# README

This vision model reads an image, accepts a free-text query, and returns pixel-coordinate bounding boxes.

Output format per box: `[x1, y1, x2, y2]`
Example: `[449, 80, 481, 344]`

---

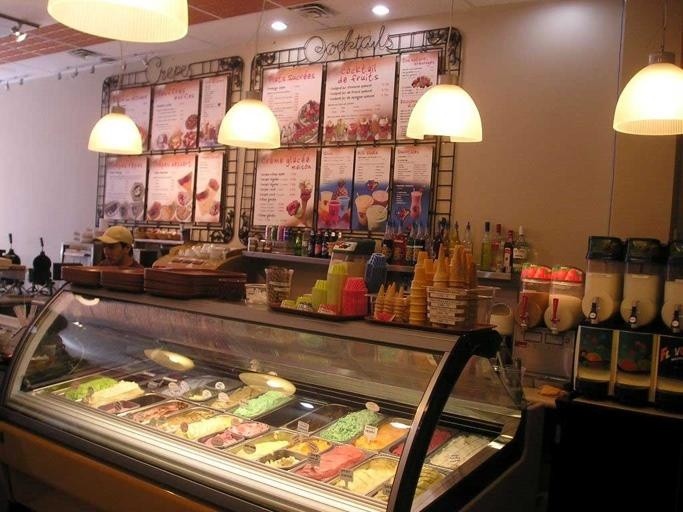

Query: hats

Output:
[92, 226, 133, 247]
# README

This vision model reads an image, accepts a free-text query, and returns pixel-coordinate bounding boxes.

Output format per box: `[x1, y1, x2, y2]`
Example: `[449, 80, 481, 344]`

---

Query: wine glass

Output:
[299, 183, 312, 223]
[178, 170, 192, 196]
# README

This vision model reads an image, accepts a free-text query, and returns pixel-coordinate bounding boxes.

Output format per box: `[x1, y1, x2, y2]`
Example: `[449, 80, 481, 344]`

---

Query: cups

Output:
[324, 115, 392, 140]
[319, 188, 349, 216]
[409, 190, 422, 219]
[264, 263, 294, 309]
[355, 190, 387, 231]
[198, 179, 219, 222]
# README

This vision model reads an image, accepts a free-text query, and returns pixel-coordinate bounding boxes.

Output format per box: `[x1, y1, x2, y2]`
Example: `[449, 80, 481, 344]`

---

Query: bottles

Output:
[379, 219, 528, 272]
[265, 222, 342, 260]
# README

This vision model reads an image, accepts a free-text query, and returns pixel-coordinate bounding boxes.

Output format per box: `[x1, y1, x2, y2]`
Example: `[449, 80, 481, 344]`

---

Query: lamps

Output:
[45, 0, 285, 158]
[10, 22, 28, 44]
[405, 2, 483, 144]
[612, 1, 683, 137]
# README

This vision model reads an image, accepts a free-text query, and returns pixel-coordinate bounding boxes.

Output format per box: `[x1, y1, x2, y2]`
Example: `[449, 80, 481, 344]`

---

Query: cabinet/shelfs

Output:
[59, 240, 104, 268]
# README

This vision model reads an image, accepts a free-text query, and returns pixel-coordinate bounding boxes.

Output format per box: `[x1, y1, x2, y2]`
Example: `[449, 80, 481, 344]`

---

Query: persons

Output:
[91, 224, 145, 270]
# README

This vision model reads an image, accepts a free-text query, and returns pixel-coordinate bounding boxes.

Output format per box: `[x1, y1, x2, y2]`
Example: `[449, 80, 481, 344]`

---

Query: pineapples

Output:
[336, 179, 349, 198]
[298, 181, 311, 213]
[285, 201, 308, 227]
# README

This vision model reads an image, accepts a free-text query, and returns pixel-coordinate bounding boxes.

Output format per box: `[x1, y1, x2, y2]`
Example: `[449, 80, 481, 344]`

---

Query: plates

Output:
[100, 200, 192, 223]
[157, 112, 198, 152]
[281, 99, 320, 145]
